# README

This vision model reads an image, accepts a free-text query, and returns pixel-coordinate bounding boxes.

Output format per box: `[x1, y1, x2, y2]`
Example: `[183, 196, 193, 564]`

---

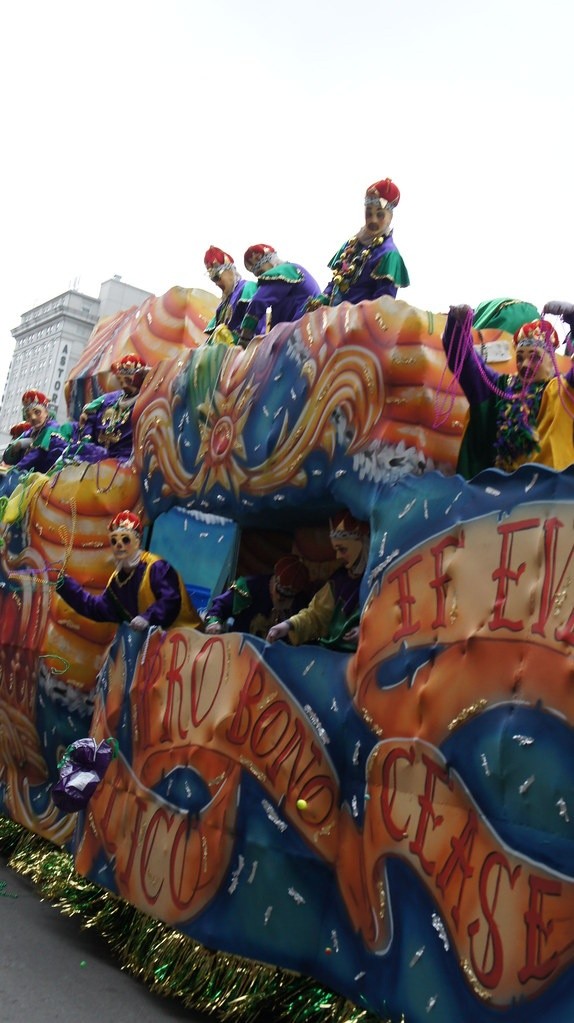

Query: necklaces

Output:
[0, 423, 129, 675]
[334, 234, 384, 293]
[106, 392, 138, 434]
[432, 308, 574, 430]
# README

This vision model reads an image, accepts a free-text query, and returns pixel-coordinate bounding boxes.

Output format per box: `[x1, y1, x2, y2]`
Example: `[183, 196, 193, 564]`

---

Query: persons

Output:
[55, 510, 205, 633]
[54, 356, 151, 465]
[306, 178, 410, 314]
[13, 392, 68, 475]
[237, 244, 321, 350]
[267, 515, 370, 655]
[203, 246, 266, 347]
[206, 554, 319, 647]
[442, 300, 574, 480]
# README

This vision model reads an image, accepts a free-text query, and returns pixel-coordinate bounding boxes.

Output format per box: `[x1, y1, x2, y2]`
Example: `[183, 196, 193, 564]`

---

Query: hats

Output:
[365, 177, 402, 212]
[514, 319, 561, 350]
[22, 390, 50, 407]
[204, 245, 235, 269]
[327, 514, 367, 541]
[273, 555, 311, 596]
[108, 511, 145, 533]
[110, 354, 145, 372]
[244, 244, 277, 272]
